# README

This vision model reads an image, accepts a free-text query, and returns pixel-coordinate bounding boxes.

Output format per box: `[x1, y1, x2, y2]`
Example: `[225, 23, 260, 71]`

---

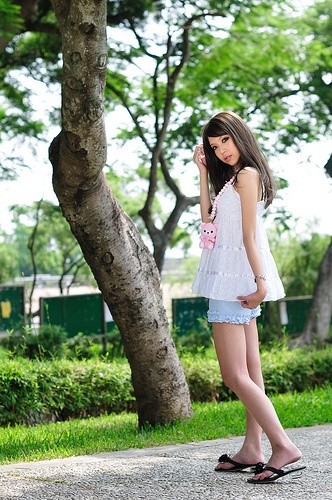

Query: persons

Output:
[192, 110, 308, 485]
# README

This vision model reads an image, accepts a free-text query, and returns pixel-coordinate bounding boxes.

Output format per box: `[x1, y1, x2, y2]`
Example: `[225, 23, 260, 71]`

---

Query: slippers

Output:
[214, 454, 266, 472]
[247, 465, 306, 483]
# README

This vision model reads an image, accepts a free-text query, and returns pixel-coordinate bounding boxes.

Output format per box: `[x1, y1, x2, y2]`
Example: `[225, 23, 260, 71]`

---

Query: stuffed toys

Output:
[199, 222, 219, 249]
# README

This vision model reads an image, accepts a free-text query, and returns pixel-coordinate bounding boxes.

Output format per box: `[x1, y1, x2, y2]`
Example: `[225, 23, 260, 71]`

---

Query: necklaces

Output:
[198, 169, 239, 249]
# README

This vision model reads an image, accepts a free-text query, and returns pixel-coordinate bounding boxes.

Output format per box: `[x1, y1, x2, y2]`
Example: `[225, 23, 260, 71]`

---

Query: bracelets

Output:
[253, 273, 267, 284]
